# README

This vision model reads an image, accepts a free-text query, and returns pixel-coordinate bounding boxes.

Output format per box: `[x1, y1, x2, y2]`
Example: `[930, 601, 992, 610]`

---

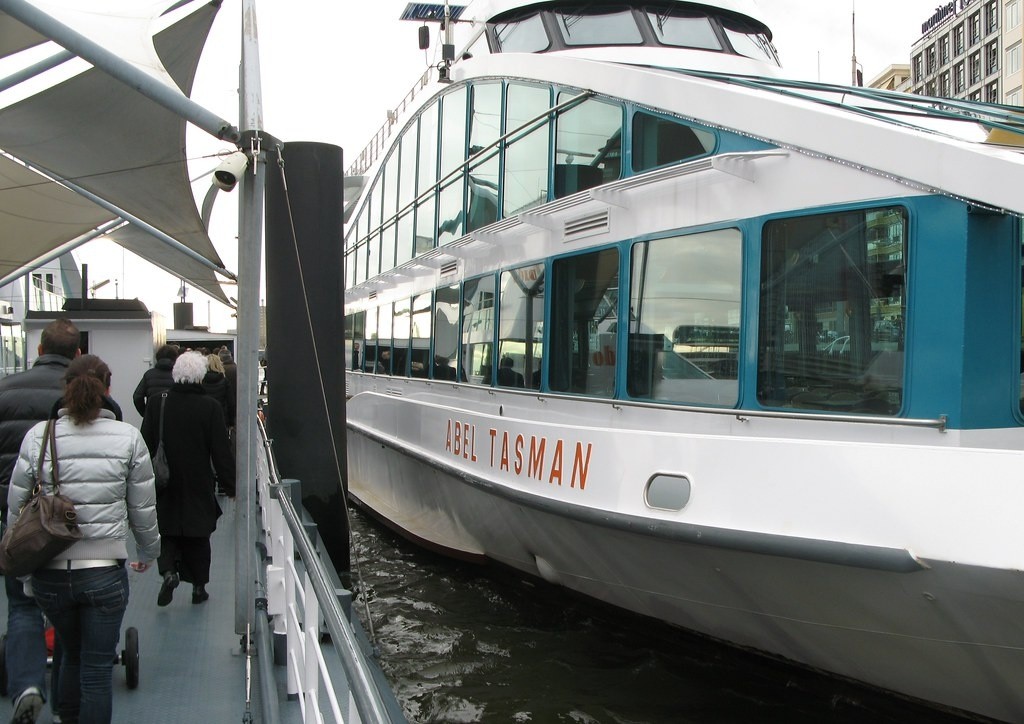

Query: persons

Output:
[4, 354, 161, 724]
[139, 351, 237, 607]
[350, 342, 542, 392]
[0, 317, 123, 724]
[133, 341, 237, 499]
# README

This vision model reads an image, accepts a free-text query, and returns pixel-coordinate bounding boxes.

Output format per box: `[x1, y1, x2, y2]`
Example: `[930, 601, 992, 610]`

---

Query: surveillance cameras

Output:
[212, 152, 249, 192]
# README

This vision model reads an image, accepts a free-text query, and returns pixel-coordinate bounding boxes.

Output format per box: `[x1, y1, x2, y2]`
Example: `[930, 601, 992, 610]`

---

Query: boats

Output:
[345, 0, 1024, 724]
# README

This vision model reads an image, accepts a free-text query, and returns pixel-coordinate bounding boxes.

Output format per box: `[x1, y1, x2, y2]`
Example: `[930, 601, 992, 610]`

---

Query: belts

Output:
[47, 559, 118, 569]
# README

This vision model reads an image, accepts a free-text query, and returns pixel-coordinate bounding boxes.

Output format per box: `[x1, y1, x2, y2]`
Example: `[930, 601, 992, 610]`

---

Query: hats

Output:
[218, 349, 231, 356]
[221, 346, 227, 350]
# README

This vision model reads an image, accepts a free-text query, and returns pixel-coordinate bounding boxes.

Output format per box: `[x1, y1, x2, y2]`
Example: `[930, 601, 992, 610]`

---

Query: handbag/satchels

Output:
[0, 419, 82, 578]
[151, 389, 171, 484]
[260, 360, 266, 366]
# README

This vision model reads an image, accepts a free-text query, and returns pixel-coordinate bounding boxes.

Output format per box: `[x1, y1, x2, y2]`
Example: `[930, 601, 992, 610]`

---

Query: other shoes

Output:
[192, 587, 209, 603]
[9, 686, 43, 724]
[158, 572, 179, 606]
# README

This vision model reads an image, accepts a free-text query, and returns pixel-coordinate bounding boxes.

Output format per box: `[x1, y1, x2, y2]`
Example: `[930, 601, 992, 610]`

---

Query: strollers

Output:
[41, 607, 142, 692]
